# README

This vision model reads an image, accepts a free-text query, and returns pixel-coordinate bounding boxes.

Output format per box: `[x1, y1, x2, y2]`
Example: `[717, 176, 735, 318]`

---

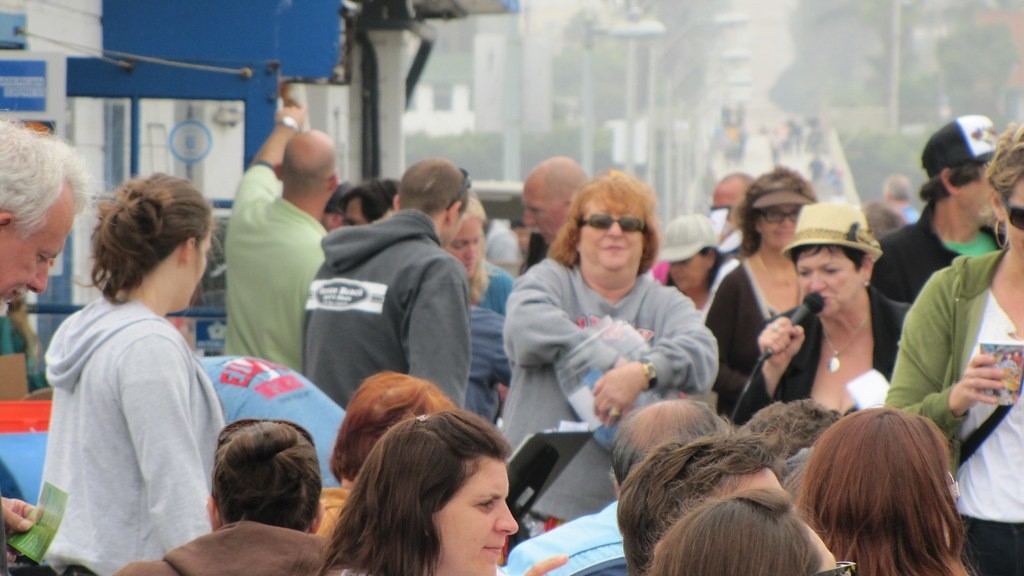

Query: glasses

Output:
[580, 213, 646, 232]
[711, 205, 731, 215]
[988, 193, 1024, 231]
[446, 168, 472, 210]
[814, 561, 858, 576]
[757, 210, 801, 222]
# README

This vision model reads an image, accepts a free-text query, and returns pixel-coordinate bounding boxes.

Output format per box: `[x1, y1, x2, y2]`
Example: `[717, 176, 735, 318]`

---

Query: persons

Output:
[190, 99, 1024, 575]
[0, 121, 86, 575]
[29, 172, 225, 576]
[111, 419, 330, 576]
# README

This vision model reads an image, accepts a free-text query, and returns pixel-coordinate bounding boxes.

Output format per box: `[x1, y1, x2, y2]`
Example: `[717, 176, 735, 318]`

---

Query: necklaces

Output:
[822, 310, 869, 372]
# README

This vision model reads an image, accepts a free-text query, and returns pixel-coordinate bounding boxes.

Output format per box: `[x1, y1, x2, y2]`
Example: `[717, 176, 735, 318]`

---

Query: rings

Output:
[608, 407, 621, 417]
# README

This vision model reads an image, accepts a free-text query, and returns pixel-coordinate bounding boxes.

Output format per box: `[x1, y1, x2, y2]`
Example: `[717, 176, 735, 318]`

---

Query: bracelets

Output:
[277, 116, 301, 132]
[640, 358, 658, 389]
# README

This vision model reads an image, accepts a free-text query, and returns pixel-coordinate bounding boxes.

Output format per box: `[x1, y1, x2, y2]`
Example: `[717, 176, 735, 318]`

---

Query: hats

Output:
[658, 214, 718, 263]
[752, 177, 811, 206]
[922, 115, 1006, 176]
[780, 201, 883, 262]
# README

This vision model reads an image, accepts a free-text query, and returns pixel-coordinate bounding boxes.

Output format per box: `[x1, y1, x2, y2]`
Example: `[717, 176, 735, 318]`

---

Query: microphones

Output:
[766, 295, 824, 355]
